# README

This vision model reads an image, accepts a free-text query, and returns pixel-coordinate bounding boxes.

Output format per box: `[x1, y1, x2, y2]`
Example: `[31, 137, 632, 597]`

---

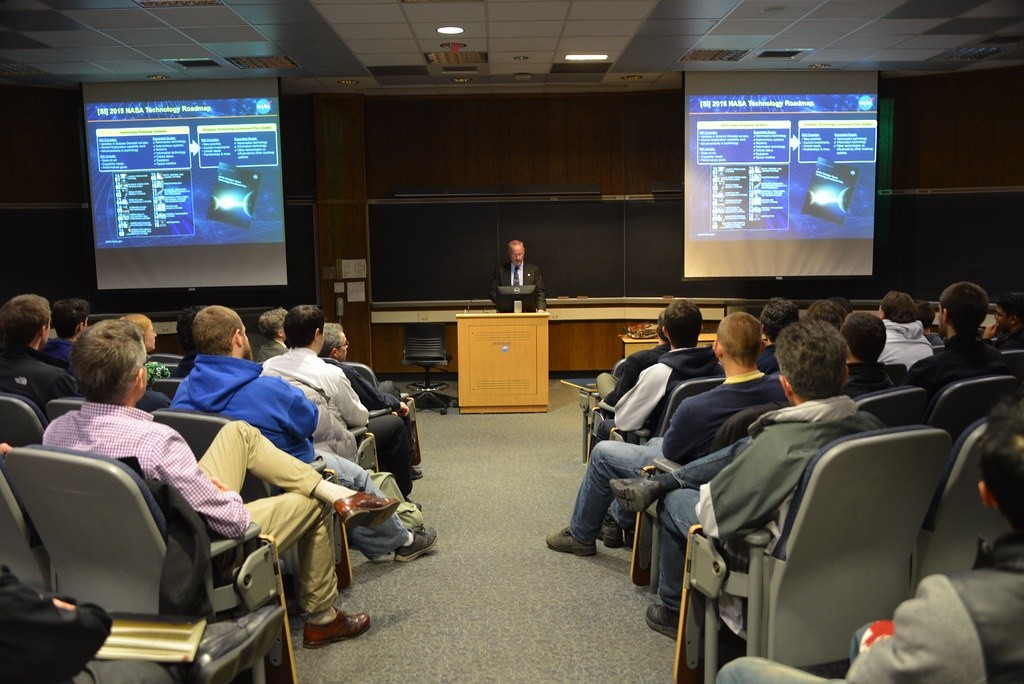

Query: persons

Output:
[546, 281, 1024, 684]
[42, 319, 400, 649]
[169, 305, 437, 563]
[0, 294, 170, 451]
[0, 564, 113, 684]
[488, 240, 546, 313]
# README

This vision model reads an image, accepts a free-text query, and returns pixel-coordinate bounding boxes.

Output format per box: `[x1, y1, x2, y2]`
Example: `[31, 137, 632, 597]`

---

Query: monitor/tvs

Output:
[497, 285, 537, 313]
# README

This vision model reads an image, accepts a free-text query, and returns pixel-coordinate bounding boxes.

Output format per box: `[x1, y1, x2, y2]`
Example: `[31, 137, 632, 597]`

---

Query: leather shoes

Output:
[334, 490, 400, 529]
[303, 606, 370, 648]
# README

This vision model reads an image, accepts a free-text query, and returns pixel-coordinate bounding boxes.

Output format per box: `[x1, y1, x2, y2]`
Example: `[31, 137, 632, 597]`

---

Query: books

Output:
[94, 610, 207, 662]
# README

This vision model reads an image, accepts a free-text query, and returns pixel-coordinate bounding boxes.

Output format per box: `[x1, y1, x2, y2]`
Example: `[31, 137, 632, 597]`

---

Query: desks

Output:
[618, 332, 771, 359]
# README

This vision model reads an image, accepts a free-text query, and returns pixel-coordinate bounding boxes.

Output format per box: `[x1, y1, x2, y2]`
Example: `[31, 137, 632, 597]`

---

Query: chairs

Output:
[563, 345, 1024, 684]
[0, 322, 453, 684]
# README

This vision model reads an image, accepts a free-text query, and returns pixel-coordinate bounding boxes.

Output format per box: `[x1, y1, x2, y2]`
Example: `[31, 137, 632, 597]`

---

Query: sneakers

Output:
[394, 524, 437, 562]
[609, 466, 661, 513]
[546, 527, 596, 556]
[646, 602, 680, 640]
[598, 516, 625, 549]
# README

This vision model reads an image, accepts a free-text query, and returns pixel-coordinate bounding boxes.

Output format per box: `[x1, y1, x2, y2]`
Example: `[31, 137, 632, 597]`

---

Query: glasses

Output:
[335, 341, 349, 348]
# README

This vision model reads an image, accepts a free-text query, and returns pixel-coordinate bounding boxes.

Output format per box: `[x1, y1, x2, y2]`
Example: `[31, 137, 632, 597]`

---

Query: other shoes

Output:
[406, 497, 422, 510]
[409, 464, 422, 479]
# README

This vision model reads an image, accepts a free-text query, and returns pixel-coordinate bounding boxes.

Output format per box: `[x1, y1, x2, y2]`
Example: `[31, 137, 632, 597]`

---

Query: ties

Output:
[513, 266, 519, 286]
